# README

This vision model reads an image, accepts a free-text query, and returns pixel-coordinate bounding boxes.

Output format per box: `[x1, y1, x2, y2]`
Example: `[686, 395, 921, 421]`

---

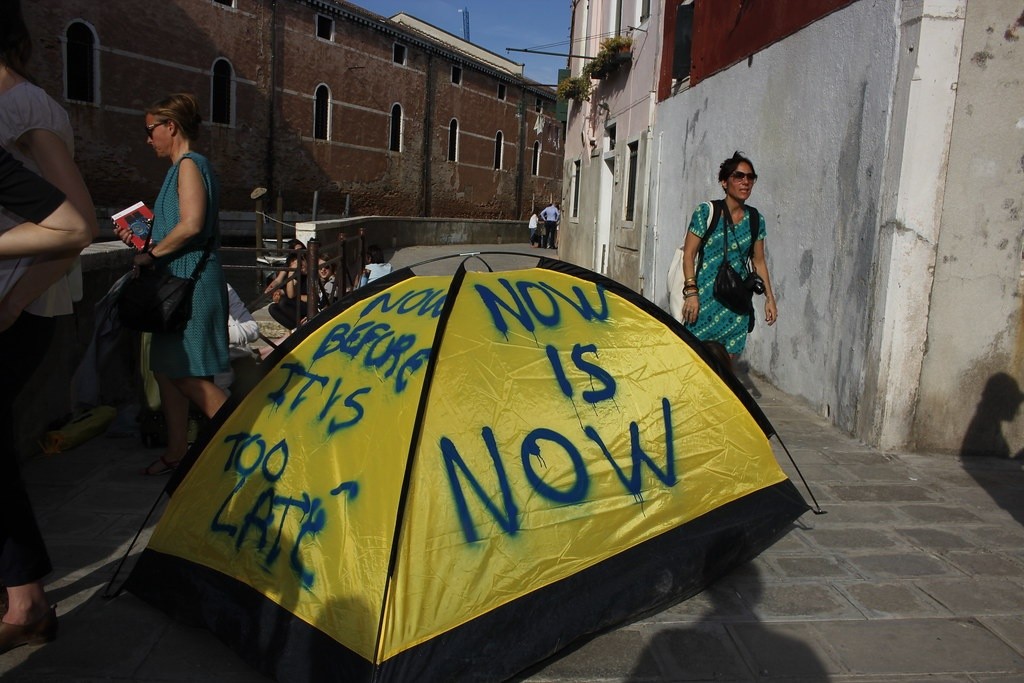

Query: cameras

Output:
[744, 272, 766, 295]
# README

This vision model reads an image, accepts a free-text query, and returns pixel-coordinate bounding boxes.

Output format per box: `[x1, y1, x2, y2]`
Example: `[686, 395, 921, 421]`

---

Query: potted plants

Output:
[555, 37, 633, 104]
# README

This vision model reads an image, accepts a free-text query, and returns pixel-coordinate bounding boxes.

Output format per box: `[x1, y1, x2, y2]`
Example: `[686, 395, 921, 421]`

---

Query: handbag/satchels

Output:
[116, 261, 192, 337]
[713, 261, 755, 314]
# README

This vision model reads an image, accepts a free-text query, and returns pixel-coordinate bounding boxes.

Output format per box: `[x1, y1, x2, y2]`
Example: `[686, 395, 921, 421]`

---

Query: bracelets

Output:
[148, 251, 155, 259]
[682, 277, 698, 300]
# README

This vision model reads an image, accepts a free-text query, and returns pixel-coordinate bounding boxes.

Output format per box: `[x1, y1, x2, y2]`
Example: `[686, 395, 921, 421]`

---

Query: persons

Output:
[540, 201, 559, 248]
[529, 210, 539, 242]
[682, 151, 777, 359]
[0, 0, 101, 652]
[265, 239, 393, 330]
[114, 93, 259, 477]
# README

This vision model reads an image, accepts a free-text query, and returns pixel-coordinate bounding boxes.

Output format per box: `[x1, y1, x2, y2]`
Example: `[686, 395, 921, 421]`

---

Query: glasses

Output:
[324, 265, 332, 269]
[145, 122, 166, 140]
[729, 171, 758, 184]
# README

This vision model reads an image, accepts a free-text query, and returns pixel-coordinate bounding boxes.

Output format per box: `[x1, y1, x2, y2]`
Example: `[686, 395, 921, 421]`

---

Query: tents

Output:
[107, 251, 819, 683]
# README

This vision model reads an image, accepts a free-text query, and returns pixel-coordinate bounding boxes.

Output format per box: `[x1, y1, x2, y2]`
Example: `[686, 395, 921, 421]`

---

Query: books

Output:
[111, 201, 154, 252]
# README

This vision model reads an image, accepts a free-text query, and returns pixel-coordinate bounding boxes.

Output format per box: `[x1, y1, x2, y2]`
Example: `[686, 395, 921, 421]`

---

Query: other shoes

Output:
[147, 455, 180, 475]
[0, 603, 56, 654]
